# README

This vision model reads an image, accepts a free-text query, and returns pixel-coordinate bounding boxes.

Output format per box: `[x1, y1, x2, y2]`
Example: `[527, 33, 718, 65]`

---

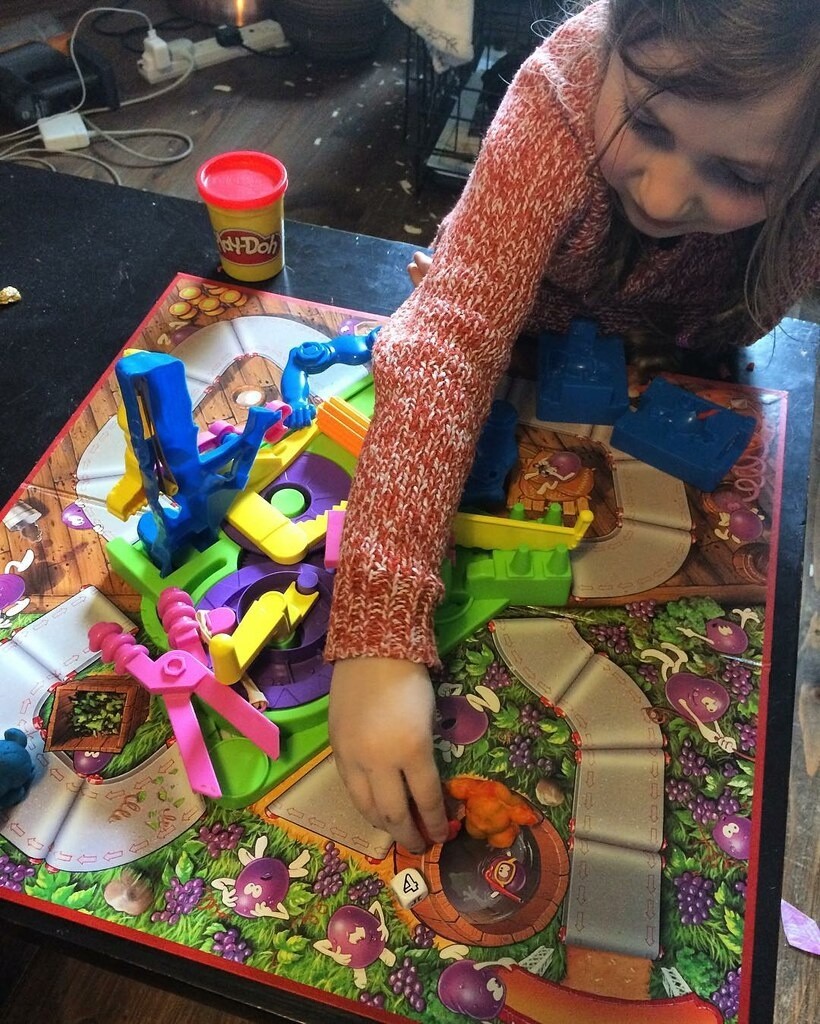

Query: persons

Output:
[317, 0, 820, 854]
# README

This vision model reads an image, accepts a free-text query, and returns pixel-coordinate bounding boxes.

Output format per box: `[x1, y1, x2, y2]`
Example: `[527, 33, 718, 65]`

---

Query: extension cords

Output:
[137, 19, 285, 84]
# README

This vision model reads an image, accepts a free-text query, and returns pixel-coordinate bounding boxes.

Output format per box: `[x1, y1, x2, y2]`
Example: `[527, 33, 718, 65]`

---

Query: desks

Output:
[0, 164, 820, 1024]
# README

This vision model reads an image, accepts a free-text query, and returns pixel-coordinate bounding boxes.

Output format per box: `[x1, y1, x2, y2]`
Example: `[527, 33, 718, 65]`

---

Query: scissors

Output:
[88, 587, 278, 800]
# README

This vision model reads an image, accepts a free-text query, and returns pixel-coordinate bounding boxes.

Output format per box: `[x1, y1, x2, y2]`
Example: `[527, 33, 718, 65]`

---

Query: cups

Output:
[196, 150, 290, 282]
